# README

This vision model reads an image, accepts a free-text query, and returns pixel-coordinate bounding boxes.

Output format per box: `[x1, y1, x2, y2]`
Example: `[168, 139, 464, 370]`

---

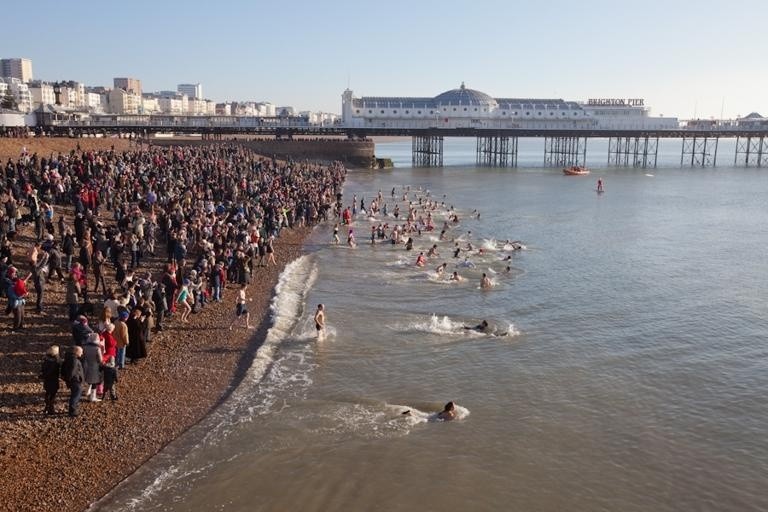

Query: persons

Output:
[333, 183, 520, 291]
[404, 402, 455, 420]
[1, 122, 349, 418]
[463, 320, 488, 331]
[596, 178, 603, 191]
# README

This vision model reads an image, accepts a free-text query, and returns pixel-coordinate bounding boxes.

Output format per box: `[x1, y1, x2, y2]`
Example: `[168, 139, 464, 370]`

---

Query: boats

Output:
[561, 167, 590, 176]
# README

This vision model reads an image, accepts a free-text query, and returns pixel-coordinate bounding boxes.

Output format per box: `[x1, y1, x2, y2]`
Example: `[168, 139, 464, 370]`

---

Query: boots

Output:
[89, 389, 102, 402]
[85, 384, 92, 396]
